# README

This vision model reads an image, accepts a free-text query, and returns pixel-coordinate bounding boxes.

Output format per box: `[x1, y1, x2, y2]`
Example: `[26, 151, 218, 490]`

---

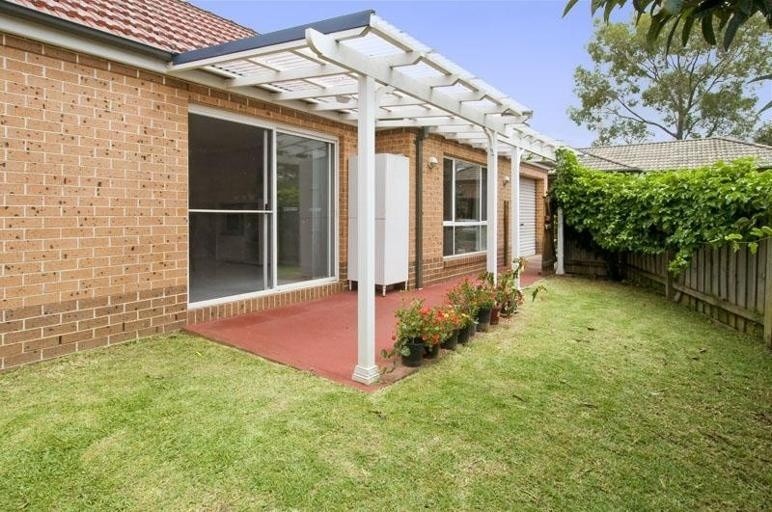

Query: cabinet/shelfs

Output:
[347, 153, 410, 296]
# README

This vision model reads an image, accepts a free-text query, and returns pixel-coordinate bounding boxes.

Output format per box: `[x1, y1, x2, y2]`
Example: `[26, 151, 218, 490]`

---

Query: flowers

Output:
[380, 257, 528, 376]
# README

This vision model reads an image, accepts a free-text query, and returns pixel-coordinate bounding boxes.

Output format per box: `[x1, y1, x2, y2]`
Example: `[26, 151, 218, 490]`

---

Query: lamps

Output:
[503, 176, 509, 186]
[427, 157, 437, 168]
